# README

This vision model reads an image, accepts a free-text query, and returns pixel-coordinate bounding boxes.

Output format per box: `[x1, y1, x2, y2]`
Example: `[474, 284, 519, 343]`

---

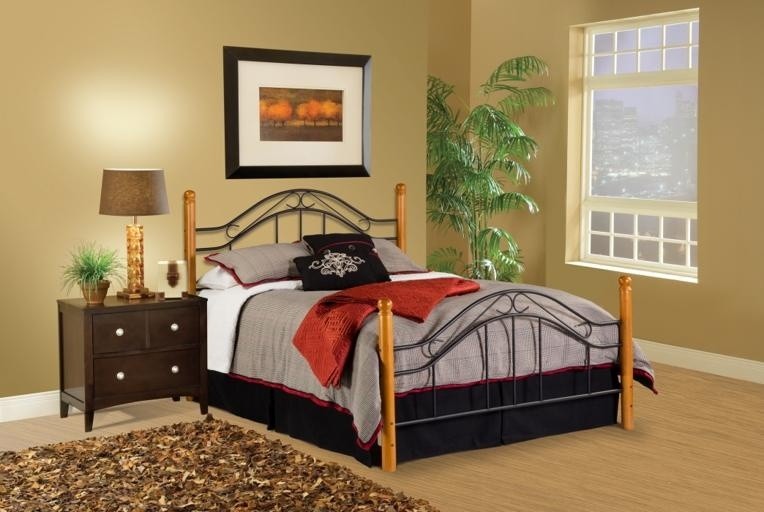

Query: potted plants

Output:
[57, 238, 133, 306]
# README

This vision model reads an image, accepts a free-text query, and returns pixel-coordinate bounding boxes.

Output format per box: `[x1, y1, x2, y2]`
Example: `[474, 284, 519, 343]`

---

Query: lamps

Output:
[99, 168, 171, 300]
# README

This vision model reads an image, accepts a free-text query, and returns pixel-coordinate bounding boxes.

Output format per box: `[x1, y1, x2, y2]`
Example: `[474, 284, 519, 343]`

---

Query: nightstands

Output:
[57, 292, 208, 432]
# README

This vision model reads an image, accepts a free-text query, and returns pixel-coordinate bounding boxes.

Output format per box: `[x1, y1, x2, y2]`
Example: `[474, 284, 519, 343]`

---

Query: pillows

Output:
[293, 249, 377, 291]
[371, 238, 428, 274]
[204, 243, 312, 288]
[195, 263, 239, 291]
[303, 233, 392, 282]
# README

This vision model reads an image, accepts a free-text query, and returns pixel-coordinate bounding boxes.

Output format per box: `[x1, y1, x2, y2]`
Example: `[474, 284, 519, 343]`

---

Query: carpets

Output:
[0, 413, 439, 512]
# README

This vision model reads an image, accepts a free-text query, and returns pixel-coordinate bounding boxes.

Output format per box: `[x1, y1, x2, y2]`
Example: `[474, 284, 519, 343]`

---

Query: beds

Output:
[184, 183, 634, 473]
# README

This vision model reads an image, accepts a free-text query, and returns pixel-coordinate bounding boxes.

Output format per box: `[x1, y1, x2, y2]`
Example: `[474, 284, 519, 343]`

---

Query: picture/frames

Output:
[223, 46, 371, 178]
